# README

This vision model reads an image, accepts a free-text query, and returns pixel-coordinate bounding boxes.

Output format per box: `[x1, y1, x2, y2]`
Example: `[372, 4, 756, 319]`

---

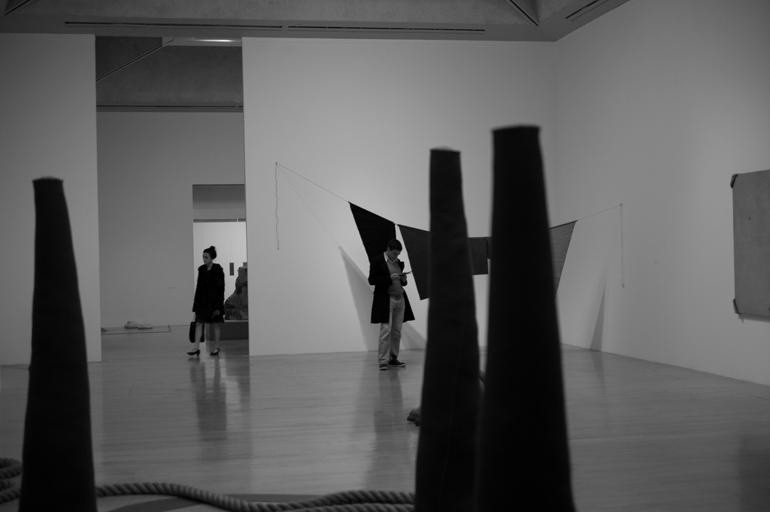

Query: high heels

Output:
[210, 347, 220, 356]
[187, 350, 200, 356]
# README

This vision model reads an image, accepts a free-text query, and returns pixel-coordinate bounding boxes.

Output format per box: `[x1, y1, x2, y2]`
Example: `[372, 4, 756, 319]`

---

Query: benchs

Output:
[205, 317, 249, 342]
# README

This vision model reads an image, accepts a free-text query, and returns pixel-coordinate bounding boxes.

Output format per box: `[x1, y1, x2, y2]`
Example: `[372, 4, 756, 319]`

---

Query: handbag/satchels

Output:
[189, 313, 205, 342]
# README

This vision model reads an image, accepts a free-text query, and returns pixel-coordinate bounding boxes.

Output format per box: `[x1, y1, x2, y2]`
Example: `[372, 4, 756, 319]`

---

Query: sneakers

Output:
[388, 359, 405, 366]
[379, 364, 388, 370]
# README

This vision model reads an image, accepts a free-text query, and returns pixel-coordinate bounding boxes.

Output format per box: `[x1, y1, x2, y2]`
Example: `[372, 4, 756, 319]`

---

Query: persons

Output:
[186, 245, 226, 360]
[366, 236, 415, 374]
